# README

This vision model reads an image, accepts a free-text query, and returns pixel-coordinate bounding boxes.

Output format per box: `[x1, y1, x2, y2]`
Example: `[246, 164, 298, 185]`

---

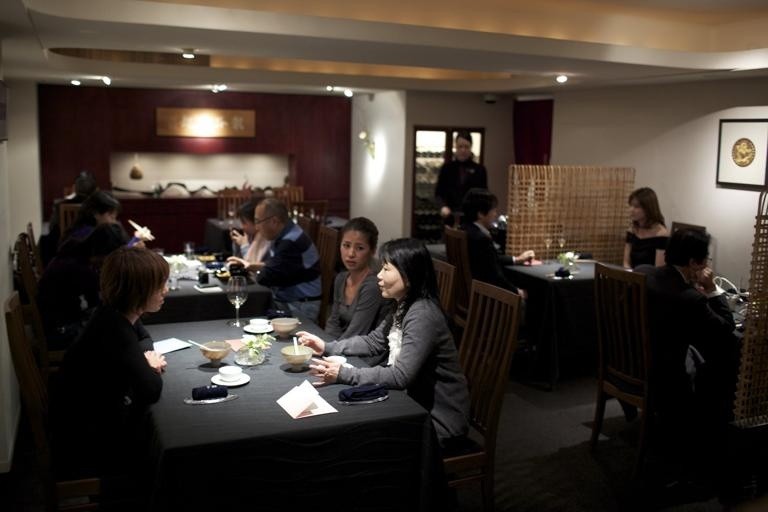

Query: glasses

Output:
[254, 216, 273, 224]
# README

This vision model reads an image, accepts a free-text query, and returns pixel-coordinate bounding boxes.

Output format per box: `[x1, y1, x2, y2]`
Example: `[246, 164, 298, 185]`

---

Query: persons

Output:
[435, 127, 489, 231]
[223, 198, 323, 325]
[36, 221, 130, 326]
[324, 216, 389, 341]
[633, 222, 738, 420]
[228, 200, 260, 267]
[459, 188, 537, 329]
[623, 186, 672, 272]
[44, 245, 172, 511]
[295, 235, 472, 454]
[59, 190, 155, 258]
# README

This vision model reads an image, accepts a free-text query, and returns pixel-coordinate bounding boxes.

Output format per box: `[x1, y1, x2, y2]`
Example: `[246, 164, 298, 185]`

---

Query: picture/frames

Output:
[716, 119, 768, 191]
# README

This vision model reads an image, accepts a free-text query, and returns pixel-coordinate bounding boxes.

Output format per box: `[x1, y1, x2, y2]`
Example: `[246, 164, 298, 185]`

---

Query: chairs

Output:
[591, 262, 684, 482]
[3, 219, 135, 511]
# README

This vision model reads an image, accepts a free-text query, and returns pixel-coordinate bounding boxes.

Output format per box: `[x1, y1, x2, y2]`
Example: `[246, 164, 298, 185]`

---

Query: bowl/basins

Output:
[272, 318, 300, 341]
[281, 346, 313, 372]
[199, 341, 231, 366]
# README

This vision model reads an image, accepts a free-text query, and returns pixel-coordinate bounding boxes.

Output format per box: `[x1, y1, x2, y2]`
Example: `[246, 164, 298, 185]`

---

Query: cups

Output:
[170, 270, 180, 291]
[329, 355, 346, 365]
[248, 320, 268, 329]
[218, 367, 241, 378]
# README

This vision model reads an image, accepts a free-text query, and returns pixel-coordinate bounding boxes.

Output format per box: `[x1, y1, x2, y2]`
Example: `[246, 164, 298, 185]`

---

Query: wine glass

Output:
[227, 202, 236, 227]
[541, 232, 567, 267]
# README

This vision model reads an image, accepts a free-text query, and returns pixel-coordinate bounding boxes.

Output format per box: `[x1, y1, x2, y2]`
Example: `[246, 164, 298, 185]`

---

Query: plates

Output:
[243, 325, 273, 334]
[211, 374, 252, 387]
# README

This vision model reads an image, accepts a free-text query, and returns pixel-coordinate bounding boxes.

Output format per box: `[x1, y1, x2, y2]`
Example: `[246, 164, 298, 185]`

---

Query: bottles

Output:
[415, 209, 443, 241]
[416, 151, 445, 183]
